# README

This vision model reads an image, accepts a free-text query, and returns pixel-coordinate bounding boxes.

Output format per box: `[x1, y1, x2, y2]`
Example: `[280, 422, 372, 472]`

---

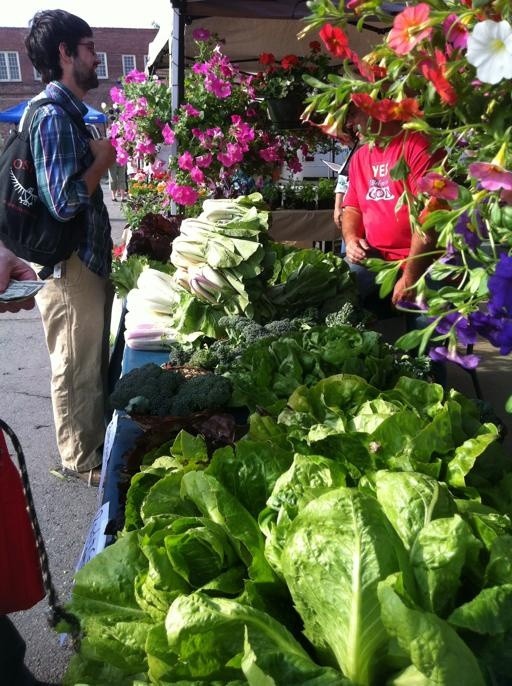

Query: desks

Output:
[74, 295, 171, 572]
[268, 208, 343, 255]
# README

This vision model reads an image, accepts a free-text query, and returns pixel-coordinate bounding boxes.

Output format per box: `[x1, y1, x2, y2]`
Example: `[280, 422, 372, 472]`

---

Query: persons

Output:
[107, 124, 129, 203]
[323, 71, 459, 353]
[331, 110, 366, 260]
[16, 7, 122, 491]
[1, 240, 40, 319]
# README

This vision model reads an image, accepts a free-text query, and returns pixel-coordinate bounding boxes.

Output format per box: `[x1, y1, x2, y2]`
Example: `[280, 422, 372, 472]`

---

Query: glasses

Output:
[79, 41, 95, 48]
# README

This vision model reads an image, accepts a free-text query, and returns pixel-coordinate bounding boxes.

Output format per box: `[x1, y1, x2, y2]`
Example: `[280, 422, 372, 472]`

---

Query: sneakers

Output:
[62, 464, 101, 486]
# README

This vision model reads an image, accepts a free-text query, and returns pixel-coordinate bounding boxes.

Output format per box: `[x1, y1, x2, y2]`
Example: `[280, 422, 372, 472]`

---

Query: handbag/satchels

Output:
[0, 97, 90, 277]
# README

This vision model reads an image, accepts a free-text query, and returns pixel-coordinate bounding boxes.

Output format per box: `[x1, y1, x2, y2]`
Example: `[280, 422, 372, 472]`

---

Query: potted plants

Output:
[271, 177, 336, 211]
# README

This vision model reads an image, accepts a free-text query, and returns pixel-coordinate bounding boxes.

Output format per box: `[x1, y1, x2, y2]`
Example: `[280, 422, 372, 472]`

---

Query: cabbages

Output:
[124, 192, 357, 352]
